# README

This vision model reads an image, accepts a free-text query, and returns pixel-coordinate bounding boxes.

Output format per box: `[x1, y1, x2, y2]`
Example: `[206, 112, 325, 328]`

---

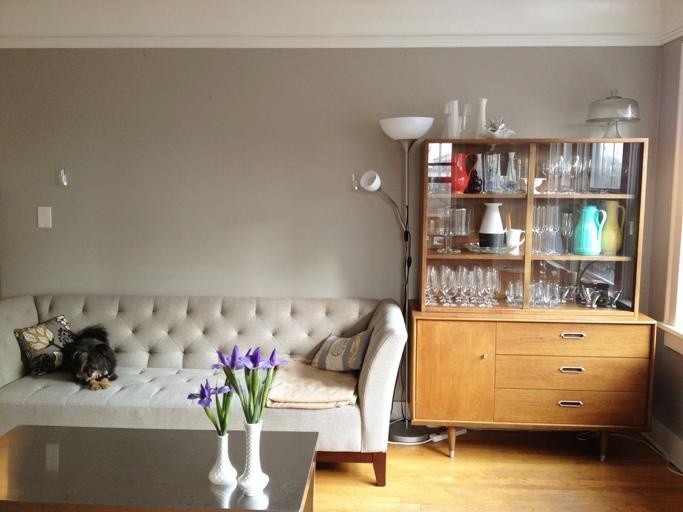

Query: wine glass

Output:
[429, 207, 470, 254]
[534, 204, 572, 256]
[542, 156, 628, 194]
[426, 264, 501, 307]
[504, 279, 577, 308]
[522, 177, 545, 194]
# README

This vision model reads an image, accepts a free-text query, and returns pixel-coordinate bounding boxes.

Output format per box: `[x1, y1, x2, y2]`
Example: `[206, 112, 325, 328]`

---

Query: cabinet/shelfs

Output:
[408, 134, 656, 464]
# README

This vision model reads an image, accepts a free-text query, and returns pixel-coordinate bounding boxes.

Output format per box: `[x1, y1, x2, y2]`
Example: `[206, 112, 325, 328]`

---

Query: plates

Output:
[464, 243, 516, 254]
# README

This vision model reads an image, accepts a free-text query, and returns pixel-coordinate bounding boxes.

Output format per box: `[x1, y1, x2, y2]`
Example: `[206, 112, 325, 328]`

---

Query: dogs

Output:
[63, 322, 117, 390]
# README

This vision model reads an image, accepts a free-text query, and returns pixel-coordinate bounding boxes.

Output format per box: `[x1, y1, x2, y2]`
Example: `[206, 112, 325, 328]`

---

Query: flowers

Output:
[186, 343, 240, 434]
[218, 344, 283, 422]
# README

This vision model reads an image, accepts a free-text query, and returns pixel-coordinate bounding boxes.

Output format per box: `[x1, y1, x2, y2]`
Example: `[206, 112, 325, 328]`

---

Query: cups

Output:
[577, 282, 621, 308]
[505, 229, 525, 254]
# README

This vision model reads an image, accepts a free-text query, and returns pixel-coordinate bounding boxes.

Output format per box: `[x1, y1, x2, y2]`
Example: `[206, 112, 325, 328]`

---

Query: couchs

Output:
[0, 290, 413, 493]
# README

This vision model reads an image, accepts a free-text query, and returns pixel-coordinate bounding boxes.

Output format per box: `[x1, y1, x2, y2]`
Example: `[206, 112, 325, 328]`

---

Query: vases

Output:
[478, 201, 506, 254]
[237, 423, 270, 495]
[202, 434, 241, 487]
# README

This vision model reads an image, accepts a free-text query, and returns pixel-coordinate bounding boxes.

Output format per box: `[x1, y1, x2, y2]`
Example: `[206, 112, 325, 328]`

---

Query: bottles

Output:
[483, 144, 500, 192]
[469, 154, 484, 193]
[442, 98, 488, 139]
[505, 152, 517, 191]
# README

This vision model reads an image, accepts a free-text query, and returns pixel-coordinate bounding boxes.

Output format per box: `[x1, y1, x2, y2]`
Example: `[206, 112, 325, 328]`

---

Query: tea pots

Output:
[573, 204, 606, 256]
[439, 150, 477, 195]
[600, 200, 626, 254]
[478, 201, 512, 248]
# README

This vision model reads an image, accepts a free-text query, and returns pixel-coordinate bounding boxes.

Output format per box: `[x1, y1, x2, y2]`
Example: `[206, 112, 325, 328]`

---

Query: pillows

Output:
[307, 328, 372, 372]
[10, 312, 79, 378]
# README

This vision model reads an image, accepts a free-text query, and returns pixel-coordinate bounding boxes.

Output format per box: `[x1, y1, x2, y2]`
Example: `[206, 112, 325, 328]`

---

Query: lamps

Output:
[356, 112, 434, 447]
